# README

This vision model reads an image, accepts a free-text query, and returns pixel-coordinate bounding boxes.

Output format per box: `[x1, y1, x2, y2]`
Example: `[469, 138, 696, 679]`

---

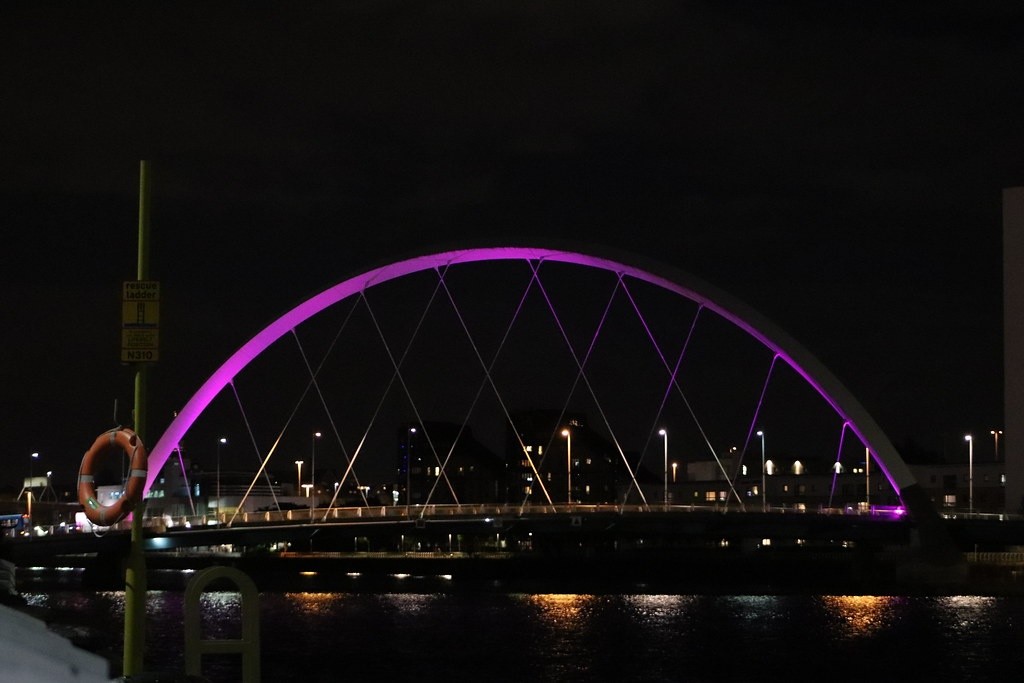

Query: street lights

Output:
[295, 461, 304, 497]
[756, 430, 766, 512]
[407, 428, 417, 520]
[217, 437, 227, 529]
[311, 433, 322, 524]
[47, 471, 52, 528]
[658, 429, 669, 513]
[29, 453, 39, 536]
[562, 430, 572, 513]
[963, 434, 974, 519]
[990, 429, 1004, 463]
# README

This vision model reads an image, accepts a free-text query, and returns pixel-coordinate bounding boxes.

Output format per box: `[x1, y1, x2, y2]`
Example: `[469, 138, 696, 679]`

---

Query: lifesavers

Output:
[77, 430, 149, 526]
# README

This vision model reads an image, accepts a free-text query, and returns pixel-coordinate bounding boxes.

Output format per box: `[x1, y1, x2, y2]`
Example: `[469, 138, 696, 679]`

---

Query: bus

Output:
[0, 514, 32, 537]
